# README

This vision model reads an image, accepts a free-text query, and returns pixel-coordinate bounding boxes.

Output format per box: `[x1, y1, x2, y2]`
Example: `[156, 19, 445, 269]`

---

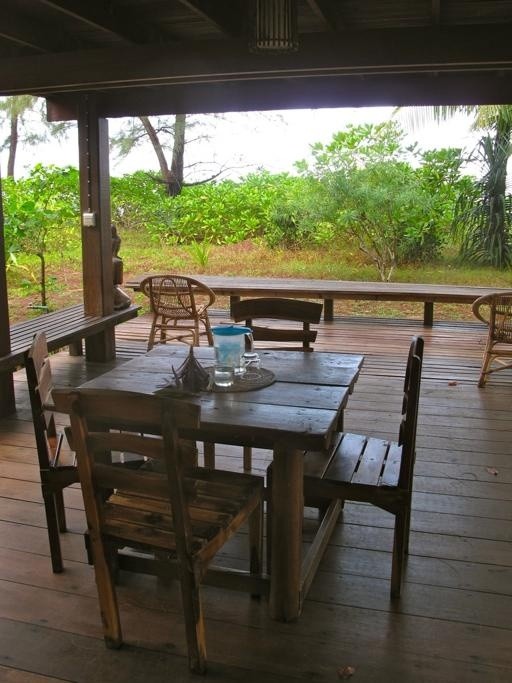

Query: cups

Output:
[213, 359, 235, 387]
[240, 353, 261, 380]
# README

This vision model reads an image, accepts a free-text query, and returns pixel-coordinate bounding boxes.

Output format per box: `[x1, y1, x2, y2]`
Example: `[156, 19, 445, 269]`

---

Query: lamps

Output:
[247, 0, 300, 56]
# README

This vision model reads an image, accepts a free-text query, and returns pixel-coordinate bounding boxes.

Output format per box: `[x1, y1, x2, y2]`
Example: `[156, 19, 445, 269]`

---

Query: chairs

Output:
[26, 332, 150, 573]
[50, 387, 264, 675]
[140, 274, 214, 353]
[232, 298, 323, 351]
[265, 334, 425, 600]
[472, 292, 512, 388]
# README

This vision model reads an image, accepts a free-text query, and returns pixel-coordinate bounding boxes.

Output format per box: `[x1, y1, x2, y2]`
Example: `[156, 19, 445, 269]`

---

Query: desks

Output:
[74, 343, 364, 622]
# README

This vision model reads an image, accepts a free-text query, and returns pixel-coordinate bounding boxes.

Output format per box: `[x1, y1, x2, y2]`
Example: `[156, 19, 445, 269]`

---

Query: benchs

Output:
[0, 305, 141, 417]
[124, 273, 512, 328]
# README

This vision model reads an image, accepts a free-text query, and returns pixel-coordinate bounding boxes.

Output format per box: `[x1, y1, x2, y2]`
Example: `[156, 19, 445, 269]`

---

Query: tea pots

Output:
[209, 326, 255, 376]
